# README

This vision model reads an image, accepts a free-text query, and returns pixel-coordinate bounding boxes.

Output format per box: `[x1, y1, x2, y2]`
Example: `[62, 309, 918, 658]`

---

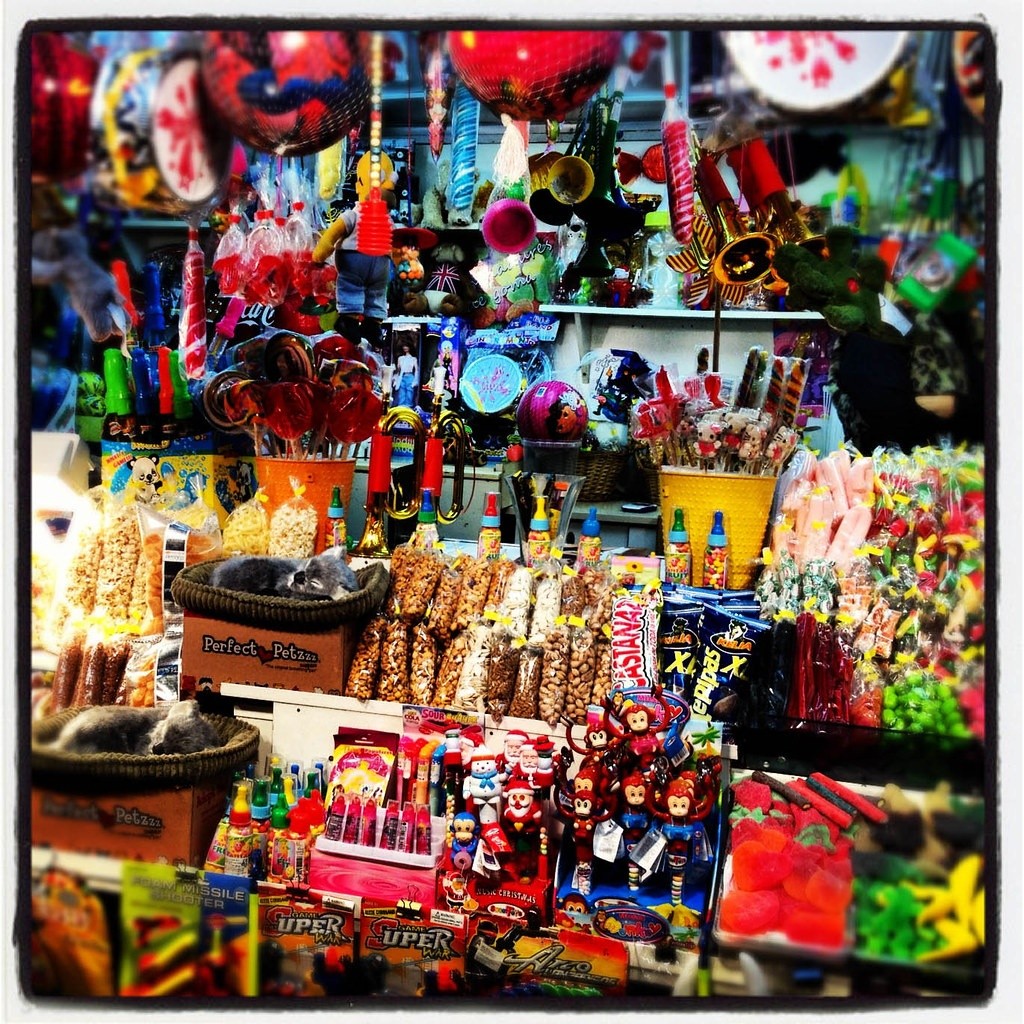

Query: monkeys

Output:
[552, 684, 723, 867]
[449, 811, 481, 862]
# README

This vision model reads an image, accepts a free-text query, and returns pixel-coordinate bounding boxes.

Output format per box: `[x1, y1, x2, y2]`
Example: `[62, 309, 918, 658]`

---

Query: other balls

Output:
[198, 29, 372, 157]
[445, 32, 624, 123]
[516, 382, 589, 442]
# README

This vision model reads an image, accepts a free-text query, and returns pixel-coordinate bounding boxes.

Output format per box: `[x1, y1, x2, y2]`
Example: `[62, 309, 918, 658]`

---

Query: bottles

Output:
[315, 479, 733, 593]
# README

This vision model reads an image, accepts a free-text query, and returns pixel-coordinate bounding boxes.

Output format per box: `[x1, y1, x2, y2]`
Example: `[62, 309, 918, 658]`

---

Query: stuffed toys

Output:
[59, 689, 221, 759]
[210, 542, 355, 602]
[436, 730, 549, 895]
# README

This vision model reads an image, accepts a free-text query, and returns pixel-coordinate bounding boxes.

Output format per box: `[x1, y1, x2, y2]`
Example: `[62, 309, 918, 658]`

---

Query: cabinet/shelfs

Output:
[34, 35, 991, 1012]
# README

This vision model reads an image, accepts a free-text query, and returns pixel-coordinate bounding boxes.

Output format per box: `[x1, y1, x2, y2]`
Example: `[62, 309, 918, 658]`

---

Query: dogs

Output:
[209, 545, 359, 602]
[50, 699, 220, 756]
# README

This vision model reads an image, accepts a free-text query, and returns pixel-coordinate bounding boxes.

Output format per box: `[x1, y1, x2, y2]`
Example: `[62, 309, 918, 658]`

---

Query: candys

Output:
[117, 316, 990, 977]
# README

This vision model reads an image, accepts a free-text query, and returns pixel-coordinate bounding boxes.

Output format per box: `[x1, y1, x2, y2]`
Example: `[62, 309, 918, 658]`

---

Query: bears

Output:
[127, 453, 163, 505]
[233, 460, 254, 504]
